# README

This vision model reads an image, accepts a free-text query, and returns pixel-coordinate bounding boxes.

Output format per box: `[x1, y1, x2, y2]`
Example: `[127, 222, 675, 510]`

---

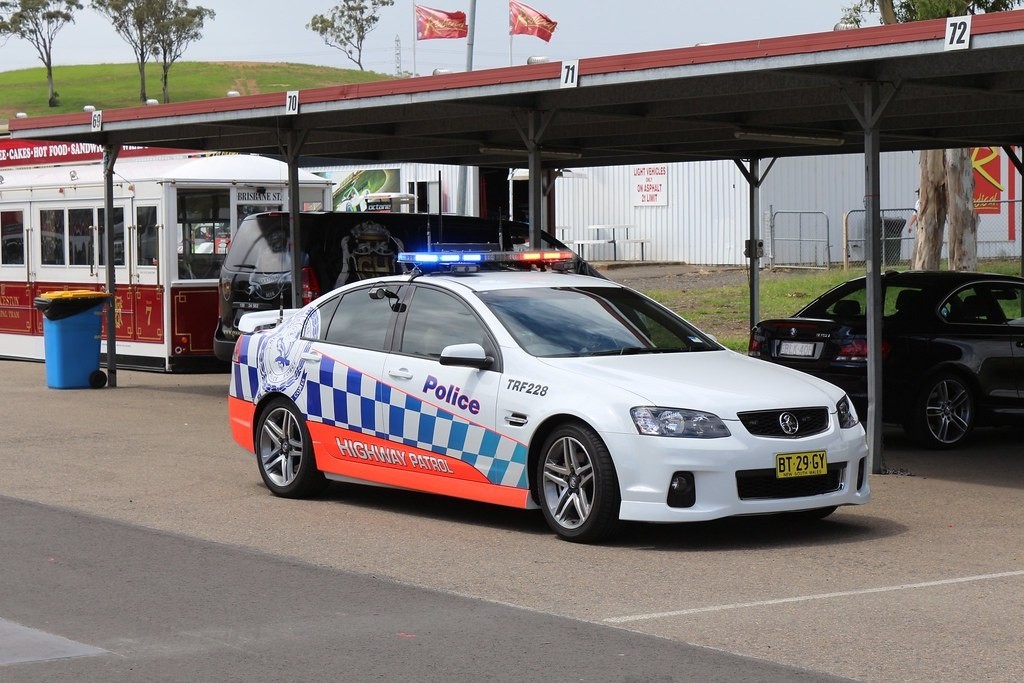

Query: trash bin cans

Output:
[33, 289, 112, 390]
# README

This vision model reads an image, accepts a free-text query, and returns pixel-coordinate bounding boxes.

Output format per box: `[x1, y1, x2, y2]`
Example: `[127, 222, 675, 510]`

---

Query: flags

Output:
[410, 4, 472, 42]
[507, 0, 562, 47]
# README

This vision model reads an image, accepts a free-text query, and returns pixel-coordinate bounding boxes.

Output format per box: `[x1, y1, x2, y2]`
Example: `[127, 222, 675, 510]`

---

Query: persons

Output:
[908, 187, 931, 270]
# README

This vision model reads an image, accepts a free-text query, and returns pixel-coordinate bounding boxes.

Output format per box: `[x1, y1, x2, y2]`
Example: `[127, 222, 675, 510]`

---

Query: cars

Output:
[747, 271, 1024, 448]
[226, 241, 870, 542]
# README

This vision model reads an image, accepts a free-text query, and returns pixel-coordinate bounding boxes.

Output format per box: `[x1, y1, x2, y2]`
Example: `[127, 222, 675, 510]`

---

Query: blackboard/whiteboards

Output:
[631, 164, 668, 206]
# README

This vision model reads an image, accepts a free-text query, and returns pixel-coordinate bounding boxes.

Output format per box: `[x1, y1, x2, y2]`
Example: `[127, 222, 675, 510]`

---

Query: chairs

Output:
[892, 289, 921, 315]
[962, 295, 987, 321]
[833, 300, 861, 314]
[190, 258, 209, 279]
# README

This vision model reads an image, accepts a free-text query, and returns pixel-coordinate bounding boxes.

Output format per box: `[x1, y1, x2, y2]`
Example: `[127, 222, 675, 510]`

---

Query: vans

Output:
[211, 208, 650, 367]
